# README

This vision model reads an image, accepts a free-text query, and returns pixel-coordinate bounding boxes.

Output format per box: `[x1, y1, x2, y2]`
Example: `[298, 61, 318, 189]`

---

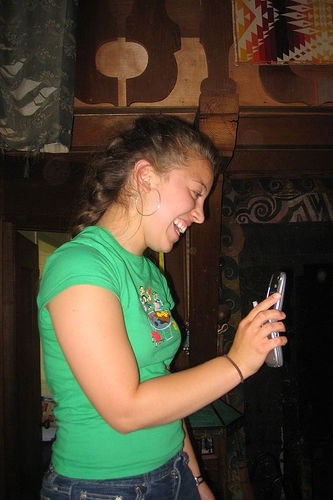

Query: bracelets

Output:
[195, 475, 204, 486]
[225, 354, 244, 384]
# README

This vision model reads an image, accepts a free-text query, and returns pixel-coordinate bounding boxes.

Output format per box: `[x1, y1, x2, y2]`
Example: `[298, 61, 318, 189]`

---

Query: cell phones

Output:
[252, 272, 287, 368]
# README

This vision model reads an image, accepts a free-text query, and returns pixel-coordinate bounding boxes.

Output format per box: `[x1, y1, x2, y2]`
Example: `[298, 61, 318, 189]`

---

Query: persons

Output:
[37, 116, 288, 500]
